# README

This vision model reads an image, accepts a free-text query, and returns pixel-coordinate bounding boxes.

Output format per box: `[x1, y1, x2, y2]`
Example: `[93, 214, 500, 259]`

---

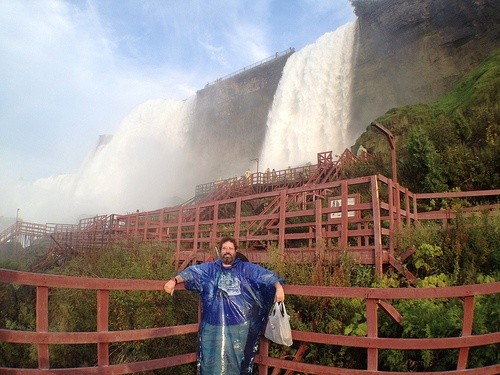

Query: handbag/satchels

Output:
[264, 301, 294, 347]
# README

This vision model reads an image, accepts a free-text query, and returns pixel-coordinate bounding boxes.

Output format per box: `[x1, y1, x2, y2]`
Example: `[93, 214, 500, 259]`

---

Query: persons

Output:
[246, 165, 310, 185]
[163, 237, 285, 375]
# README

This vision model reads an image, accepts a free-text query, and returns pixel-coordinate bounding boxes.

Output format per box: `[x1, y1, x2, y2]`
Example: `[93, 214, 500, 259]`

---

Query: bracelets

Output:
[171, 277, 177, 284]
